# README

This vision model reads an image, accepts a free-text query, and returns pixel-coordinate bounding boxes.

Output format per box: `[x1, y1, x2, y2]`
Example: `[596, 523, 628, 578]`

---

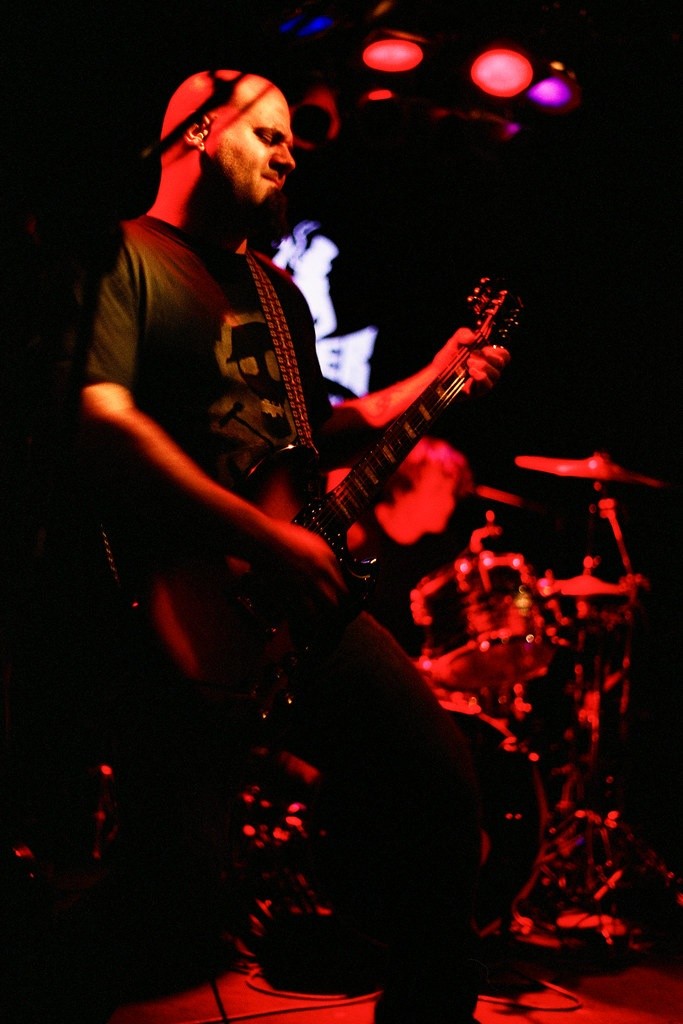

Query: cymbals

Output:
[545, 574, 627, 599]
[512, 453, 672, 489]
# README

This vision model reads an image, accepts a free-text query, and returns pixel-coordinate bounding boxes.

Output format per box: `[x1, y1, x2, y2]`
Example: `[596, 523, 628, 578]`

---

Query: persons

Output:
[1, 72, 510, 1024]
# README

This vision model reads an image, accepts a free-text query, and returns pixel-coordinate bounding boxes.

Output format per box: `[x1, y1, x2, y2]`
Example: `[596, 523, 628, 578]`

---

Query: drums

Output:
[416, 551, 550, 690]
[226, 699, 545, 948]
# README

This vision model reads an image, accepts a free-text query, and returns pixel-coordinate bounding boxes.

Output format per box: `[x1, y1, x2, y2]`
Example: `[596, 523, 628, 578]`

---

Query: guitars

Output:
[137, 270, 525, 693]
